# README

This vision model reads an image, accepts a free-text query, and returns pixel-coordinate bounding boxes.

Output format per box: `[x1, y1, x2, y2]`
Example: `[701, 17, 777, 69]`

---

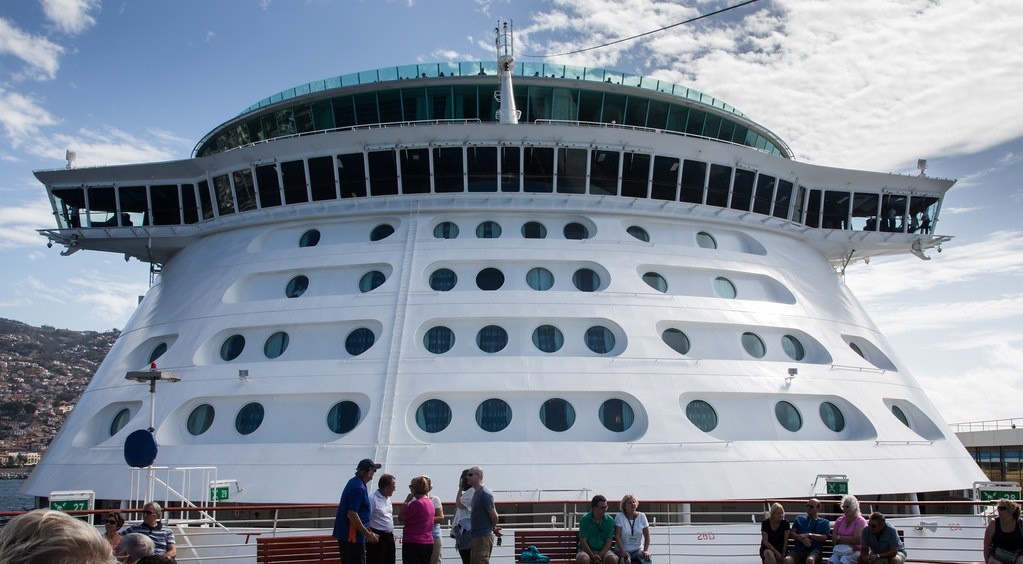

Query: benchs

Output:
[514, 530, 616, 564]
[786, 528, 904, 564]
[256, 535, 342, 564]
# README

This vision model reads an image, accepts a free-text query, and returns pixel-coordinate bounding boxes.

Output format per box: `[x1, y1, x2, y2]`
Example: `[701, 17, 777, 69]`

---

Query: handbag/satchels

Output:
[833, 544, 854, 555]
[520, 546, 550, 564]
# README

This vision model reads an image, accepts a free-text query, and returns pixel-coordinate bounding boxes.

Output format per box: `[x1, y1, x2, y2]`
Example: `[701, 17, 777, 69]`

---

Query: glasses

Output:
[467, 473, 478, 477]
[842, 505, 850, 508]
[997, 507, 1011, 511]
[143, 510, 158, 515]
[597, 506, 607, 509]
[807, 504, 814, 509]
[868, 523, 881, 528]
[106, 518, 119, 525]
[409, 485, 413, 489]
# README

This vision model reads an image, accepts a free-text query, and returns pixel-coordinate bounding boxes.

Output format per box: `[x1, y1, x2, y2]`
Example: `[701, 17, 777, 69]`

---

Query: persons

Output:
[333, 459, 396, 564]
[104, 502, 177, 564]
[827, 495, 908, 564]
[760, 499, 830, 564]
[0, 509, 116, 564]
[450, 467, 503, 564]
[398, 476, 445, 564]
[882, 206, 930, 234]
[983, 500, 1023, 564]
[576, 494, 653, 564]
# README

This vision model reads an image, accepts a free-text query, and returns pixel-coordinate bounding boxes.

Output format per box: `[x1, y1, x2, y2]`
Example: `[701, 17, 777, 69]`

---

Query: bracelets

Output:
[877, 553, 880, 559]
[837, 535, 840, 540]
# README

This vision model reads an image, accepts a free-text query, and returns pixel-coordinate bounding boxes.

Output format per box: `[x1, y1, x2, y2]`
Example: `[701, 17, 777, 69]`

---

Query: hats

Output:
[356, 458, 381, 472]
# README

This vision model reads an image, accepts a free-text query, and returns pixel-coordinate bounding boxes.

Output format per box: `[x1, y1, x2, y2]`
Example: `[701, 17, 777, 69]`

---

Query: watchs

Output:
[809, 533, 812, 538]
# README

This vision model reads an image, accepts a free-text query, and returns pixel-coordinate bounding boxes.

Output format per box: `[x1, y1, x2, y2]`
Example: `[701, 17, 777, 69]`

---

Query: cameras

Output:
[497, 537, 502, 545]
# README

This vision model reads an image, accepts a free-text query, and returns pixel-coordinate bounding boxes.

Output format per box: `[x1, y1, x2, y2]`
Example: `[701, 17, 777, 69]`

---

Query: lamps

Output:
[917, 159, 928, 178]
[785, 368, 797, 382]
[239, 369, 250, 382]
[65, 149, 76, 170]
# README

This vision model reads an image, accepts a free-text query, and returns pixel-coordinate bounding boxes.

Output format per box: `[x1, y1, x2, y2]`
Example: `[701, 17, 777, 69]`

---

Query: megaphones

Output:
[920, 522, 937, 532]
